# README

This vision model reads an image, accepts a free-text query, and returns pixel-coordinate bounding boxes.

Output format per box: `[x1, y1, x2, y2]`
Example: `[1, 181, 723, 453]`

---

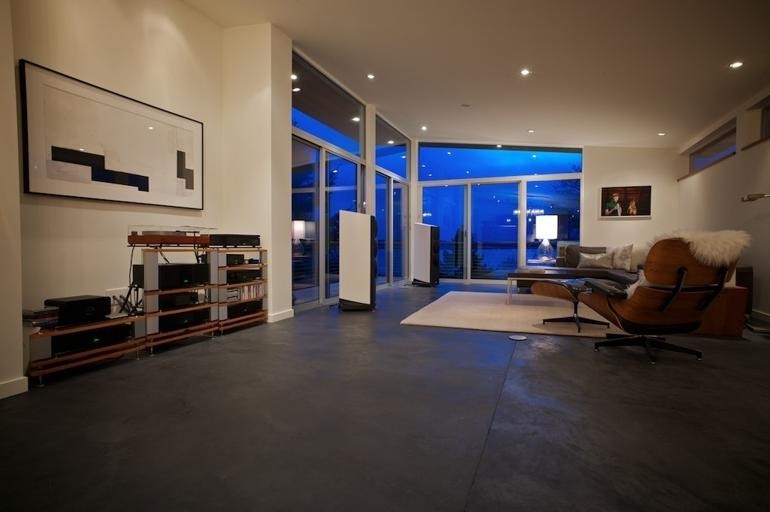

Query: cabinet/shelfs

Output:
[141, 246, 221, 357]
[214, 243, 270, 338]
[22, 307, 147, 388]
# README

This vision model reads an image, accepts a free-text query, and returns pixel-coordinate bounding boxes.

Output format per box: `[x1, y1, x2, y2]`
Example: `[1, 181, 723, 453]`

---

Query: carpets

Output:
[396, 287, 663, 346]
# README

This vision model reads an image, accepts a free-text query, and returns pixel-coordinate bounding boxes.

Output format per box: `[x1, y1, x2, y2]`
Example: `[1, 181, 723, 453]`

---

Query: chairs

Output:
[575, 223, 753, 368]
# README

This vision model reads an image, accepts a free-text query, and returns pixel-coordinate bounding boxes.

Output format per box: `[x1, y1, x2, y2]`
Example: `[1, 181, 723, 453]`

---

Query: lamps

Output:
[534, 212, 560, 262]
[742, 193, 770, 203]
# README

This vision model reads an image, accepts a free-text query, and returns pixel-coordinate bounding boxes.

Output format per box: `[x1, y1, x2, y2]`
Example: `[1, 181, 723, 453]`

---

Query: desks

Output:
[527, 256, 558, 265]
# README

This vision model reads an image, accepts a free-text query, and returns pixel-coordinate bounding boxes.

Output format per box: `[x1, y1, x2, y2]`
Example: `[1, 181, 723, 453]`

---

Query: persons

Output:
[604, 192, 622, 216]
[627, 201, 637, 215]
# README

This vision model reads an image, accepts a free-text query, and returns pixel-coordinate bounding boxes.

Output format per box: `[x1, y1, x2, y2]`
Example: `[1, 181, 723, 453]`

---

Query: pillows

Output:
[630, 244, 651, 270]
[605, 240, 634, 272]
[577, 251, 615, 270]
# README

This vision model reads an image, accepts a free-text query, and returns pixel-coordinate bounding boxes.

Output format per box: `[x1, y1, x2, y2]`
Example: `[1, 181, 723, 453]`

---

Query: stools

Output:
[530, 278, 624, 332]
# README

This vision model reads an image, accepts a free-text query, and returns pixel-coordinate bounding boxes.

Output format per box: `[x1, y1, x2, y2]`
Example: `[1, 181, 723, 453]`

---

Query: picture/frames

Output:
[17, 57, 208, 214]
[598, 183, 655, 223]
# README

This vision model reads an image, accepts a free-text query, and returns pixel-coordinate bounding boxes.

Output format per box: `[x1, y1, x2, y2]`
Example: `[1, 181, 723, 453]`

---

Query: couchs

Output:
[564, 242, 755, 342]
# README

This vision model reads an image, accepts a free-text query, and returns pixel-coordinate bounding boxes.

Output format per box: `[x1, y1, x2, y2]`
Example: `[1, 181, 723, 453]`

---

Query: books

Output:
[240, 284, 266, 300]
